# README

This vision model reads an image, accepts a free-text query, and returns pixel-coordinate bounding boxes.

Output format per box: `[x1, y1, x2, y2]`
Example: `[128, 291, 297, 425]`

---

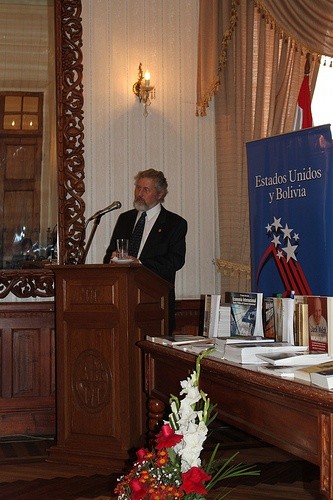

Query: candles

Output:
[145, 71, 150, 86]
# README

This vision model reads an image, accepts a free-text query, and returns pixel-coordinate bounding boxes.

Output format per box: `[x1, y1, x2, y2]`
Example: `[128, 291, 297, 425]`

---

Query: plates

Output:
[111, 259, 134, 264]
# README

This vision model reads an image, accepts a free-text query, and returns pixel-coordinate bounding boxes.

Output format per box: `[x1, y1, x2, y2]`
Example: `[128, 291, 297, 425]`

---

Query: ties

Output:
[128, 212, 146, 258]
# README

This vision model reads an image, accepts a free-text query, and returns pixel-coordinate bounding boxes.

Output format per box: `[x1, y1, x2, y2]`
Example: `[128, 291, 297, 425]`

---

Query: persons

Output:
[308, 298, 326, 329]
[102, 170, 188, 333]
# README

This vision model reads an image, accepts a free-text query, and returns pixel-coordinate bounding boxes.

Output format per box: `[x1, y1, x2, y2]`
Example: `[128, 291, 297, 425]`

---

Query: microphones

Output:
[86, 201, 122, 221]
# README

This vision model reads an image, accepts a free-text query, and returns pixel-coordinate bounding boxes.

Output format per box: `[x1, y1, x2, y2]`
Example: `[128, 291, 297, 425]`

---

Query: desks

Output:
[137, 339, 333, 500]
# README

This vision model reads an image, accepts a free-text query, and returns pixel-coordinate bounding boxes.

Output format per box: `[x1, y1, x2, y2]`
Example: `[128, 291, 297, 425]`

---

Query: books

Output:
[146, 292, 333, 389]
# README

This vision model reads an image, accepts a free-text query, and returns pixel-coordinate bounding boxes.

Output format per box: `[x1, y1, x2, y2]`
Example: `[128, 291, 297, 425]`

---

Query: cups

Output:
[116, 239, 128, 260]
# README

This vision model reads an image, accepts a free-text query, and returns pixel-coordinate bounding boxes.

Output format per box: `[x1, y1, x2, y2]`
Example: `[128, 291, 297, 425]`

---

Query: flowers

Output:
[114, 349, 261, 500]
